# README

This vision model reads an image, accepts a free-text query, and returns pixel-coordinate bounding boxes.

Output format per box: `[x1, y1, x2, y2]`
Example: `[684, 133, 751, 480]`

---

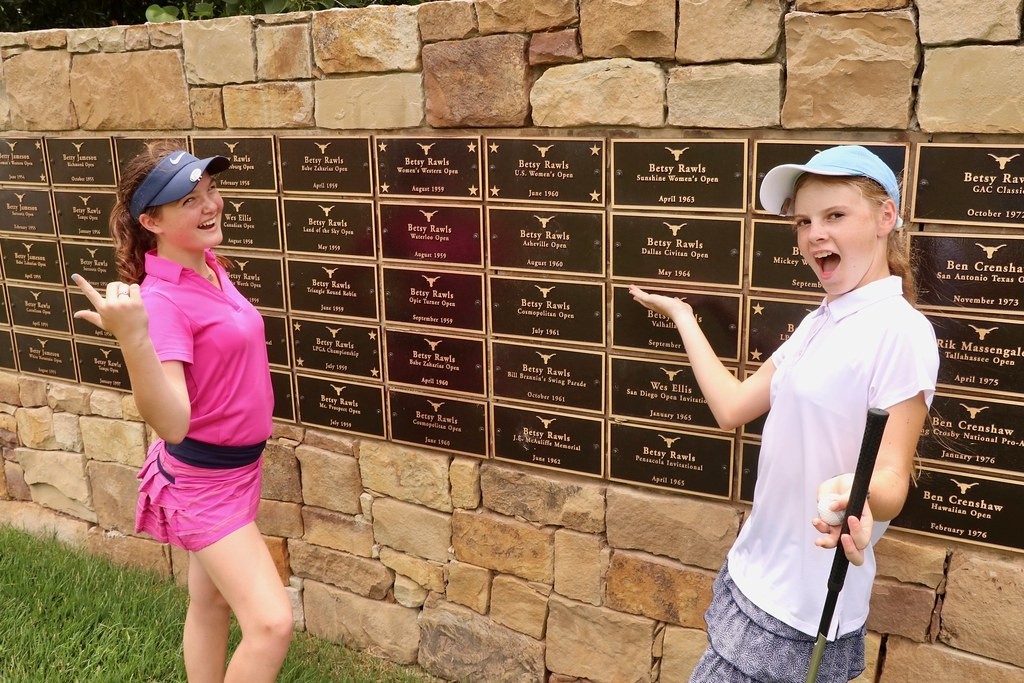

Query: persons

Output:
[70, 136, 294, 683]
[627, 145, 940, 683]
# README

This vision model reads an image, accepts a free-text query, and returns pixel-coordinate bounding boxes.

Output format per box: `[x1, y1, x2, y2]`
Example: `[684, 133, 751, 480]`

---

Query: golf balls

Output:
[817, 493, 846, 526]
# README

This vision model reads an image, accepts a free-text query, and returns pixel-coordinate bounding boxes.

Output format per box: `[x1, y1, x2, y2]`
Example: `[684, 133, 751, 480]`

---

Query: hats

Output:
[759, 145, 904, 228]
[129, 150, 230, 224]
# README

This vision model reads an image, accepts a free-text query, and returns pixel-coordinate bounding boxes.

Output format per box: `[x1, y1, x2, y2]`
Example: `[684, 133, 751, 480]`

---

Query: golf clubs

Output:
[805, 405, 891, 682]
